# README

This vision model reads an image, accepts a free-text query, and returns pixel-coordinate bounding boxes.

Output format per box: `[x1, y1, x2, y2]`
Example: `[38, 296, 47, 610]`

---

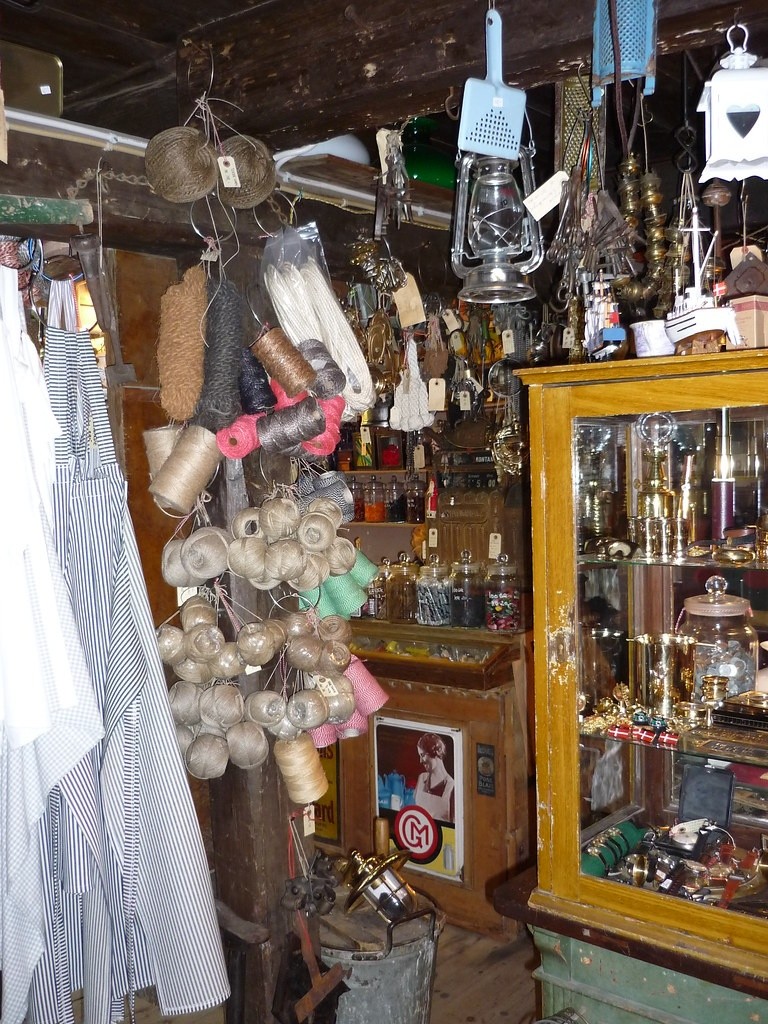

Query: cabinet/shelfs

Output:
[313, 411, 540, 942]
[511, 347, 768, 983]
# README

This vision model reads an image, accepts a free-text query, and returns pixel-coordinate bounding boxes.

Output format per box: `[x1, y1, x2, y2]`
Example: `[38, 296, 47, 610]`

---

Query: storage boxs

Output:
[725, 295, 768, 350]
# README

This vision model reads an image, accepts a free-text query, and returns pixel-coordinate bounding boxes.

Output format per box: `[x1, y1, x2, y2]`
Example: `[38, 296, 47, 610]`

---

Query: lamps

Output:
[452, 108, 545, 304]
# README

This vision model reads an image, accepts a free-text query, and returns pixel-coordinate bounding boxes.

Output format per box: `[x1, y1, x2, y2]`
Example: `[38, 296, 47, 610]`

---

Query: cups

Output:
[630, 320, 676, 357]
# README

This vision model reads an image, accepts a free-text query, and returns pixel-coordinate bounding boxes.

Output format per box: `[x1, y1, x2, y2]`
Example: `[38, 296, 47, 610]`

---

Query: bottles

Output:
[467, 175, 524, 253]
